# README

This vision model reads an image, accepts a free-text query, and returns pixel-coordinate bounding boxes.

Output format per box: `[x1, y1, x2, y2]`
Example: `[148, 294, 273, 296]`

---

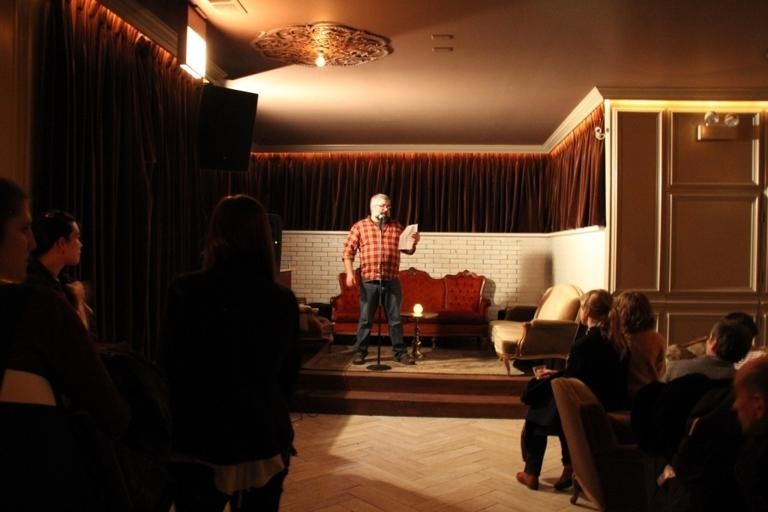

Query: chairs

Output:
[488, 282, 585, 375]
[550, 376, 642, 512]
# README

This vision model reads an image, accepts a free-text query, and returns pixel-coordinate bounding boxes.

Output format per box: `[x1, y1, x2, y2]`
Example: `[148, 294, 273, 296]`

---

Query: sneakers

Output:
[353, 354, 365, 365]
[394, 353, 414, 365]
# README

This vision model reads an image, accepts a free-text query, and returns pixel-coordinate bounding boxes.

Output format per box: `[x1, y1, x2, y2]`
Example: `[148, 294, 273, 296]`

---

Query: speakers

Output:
[198, 85, 258, 171]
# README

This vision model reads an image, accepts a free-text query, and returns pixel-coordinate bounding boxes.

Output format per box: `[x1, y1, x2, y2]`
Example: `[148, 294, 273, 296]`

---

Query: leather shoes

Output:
[554, 470, 573, 490]
[515, 471, 540, 490]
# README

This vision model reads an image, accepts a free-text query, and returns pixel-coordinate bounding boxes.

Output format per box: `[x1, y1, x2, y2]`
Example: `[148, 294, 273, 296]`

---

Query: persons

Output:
[163, 193, 299, 512]
[342, 193, 420, 365]
[0, 179, 133, 512]
[26, 210, 89, 333]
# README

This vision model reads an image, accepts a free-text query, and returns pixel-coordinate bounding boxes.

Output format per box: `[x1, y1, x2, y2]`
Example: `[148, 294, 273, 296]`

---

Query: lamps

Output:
[313, 51, 326, 66]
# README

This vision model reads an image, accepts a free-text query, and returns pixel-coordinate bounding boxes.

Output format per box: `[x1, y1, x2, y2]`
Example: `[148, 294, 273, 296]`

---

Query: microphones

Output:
[377, 213, 391, 223]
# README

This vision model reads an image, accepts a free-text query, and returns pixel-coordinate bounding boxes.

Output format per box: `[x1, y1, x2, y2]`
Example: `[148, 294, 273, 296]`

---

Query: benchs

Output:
[328, 266, 490, 354]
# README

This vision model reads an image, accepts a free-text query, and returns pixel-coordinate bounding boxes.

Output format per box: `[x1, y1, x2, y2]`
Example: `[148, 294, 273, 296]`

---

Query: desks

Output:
[399, 312, 440, 360]
